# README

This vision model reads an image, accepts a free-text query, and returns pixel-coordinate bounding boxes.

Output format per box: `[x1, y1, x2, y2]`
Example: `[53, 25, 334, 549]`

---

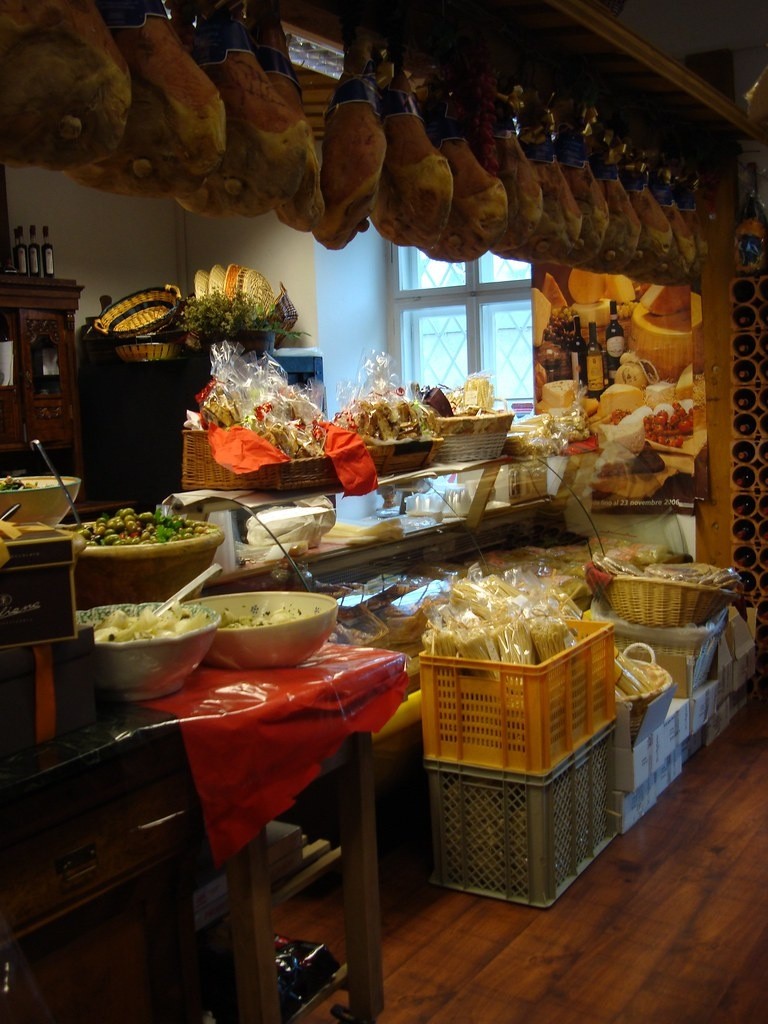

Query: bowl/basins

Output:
[76, 602, 221, 702]
[405, 479, 478, 514]
[0, 476, 82, 528]
[182, 591, 339, 668]
[62, 521, 225, 611]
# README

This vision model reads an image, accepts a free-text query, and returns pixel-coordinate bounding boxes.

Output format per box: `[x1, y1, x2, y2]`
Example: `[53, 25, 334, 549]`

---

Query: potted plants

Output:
[176, 292, 307, 355]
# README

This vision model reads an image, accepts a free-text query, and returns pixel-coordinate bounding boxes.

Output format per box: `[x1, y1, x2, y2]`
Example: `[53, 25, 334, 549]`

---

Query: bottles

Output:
[571, 315, 588, 388]
[606, 300, 624, 385]
[587, 322, 606, 401]
[12, 228, 18, 269]
[41, 226, 55, 278]
[28, 225, 40, 278]
[16, 226, 28, 276]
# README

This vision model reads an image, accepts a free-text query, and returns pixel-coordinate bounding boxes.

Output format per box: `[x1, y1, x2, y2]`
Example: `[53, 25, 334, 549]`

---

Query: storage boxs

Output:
[0, 625, 99, 759]
[608, 605, 761, 835]
[418, 618, 618, 777]
[0, 520, 78, 649]
[423, 720, 619, 909]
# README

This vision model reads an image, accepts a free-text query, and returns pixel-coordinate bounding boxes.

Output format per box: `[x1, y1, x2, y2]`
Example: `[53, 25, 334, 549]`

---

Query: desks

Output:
[143, 642, 409, 1023]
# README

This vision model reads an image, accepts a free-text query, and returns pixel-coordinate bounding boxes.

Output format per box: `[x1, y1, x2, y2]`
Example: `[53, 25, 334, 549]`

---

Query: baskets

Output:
[602, 573, 739, 628]
[93, 263, 298, 364]
[363, 438, 445, 476]
[433, 414, 517, 465]
[181, 428, 363, 492]
[613, 644, 672, 740]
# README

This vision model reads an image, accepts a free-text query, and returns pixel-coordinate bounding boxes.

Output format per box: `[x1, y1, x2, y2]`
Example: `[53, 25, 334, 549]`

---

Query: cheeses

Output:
[531, 263, 692, 413]
[584, 403, 680, 499]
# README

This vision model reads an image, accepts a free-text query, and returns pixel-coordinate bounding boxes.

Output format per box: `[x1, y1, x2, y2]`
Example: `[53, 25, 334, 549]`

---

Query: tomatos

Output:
[611, 401, 693, 447]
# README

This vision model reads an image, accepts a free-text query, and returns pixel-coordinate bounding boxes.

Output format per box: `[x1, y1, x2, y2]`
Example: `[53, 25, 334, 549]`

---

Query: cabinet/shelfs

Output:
[82, 347, 324, 506]
[0, 275, 136, 522]
[0, 699, 200, 1024]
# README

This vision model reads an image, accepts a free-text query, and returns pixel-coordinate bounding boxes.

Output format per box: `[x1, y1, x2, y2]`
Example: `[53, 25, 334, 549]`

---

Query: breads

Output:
[203, 394, 440, 460]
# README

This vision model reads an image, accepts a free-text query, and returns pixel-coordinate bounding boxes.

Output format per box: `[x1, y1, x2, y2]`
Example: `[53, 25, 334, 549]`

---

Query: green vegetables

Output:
[153, 507, 187, 542]
